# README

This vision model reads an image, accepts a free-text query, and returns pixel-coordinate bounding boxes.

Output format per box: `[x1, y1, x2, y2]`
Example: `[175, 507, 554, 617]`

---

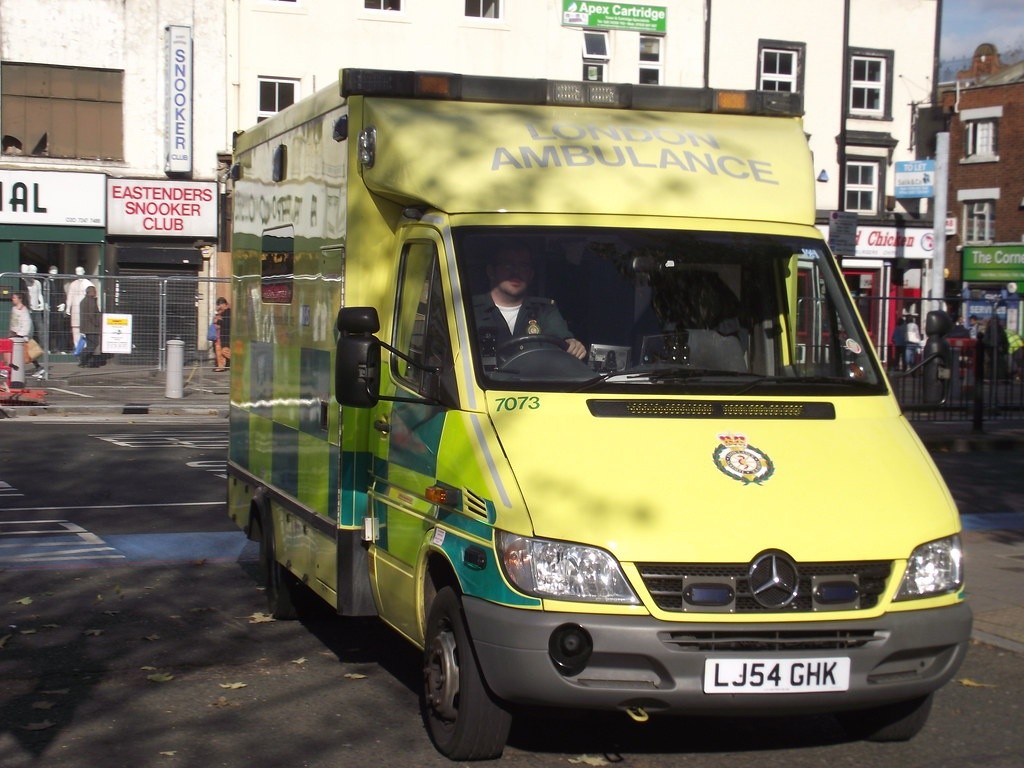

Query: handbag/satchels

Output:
[22, 338, 44, 365]
[73, 335, 87, 356]
[207, 320, 217, 341]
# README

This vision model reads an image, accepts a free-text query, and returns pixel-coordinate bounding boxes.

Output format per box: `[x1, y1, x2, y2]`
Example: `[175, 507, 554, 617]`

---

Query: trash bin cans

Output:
[11, 337, 25, 387]
[946, 339, 978, 399]
[0, 339, 13, 388]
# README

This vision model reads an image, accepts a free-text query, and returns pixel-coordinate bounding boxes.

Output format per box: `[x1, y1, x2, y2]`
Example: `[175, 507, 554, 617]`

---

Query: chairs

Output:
[662, 284, 745, 374]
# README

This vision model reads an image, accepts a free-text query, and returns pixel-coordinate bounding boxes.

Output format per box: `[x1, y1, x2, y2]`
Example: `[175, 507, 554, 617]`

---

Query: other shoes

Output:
[213, 367, 226, 372]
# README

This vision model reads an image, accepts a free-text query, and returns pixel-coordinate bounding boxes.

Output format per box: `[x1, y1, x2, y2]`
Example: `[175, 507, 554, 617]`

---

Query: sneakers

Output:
[32, 368, 45, 377]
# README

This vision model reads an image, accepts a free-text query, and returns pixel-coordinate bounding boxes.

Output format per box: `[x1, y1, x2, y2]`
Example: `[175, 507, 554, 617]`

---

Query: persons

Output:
[472, 239, 586, 359]
[212, 297, 231, 371]
[75, 286, 101, 368]
[968, 314, 1024, 381]
[10, 292, 44, 376]
[892, 315, 921, 371]
[21, 265, 95, 354]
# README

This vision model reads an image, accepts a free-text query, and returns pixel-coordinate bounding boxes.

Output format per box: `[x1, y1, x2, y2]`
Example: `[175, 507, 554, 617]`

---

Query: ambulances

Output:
[225, 65, 973, 762]
